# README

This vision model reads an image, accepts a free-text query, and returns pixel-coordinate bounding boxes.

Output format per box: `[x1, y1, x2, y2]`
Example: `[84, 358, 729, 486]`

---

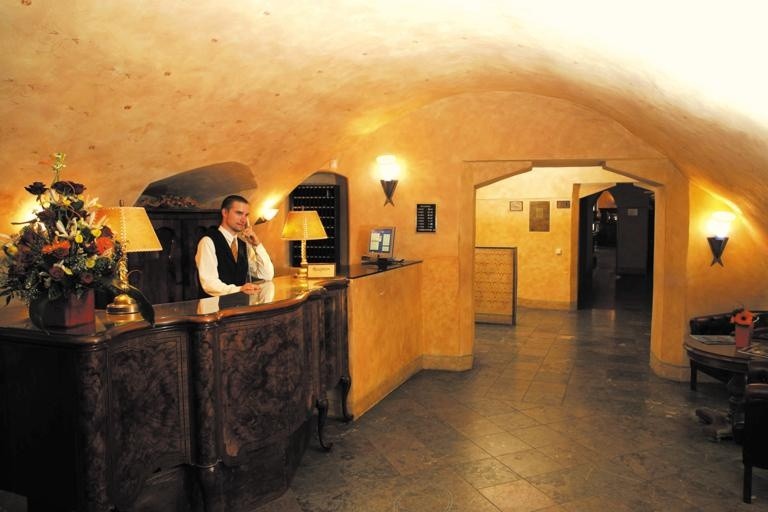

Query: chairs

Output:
[742, 360, 768, 504]
[683, 309, 768, 391]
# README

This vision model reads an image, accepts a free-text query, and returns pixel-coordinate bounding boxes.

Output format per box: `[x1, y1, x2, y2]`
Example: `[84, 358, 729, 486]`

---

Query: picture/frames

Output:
[510, 201, 523, 212]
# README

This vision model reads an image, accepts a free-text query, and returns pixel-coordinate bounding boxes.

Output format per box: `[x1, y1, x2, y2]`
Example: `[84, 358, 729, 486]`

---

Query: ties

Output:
[231, 238, 238, 264]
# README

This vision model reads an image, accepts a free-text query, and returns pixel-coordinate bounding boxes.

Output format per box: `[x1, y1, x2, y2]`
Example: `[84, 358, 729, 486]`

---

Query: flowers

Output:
[0, 152, 156, 327]
[729, 302, 760, 324]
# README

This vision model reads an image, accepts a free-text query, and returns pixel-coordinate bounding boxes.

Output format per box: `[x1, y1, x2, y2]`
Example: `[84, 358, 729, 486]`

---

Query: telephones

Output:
[245, 216, 252, 241]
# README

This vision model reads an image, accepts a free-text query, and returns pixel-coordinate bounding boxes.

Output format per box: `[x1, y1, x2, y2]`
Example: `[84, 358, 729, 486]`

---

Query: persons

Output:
[193, 195, 274, 299]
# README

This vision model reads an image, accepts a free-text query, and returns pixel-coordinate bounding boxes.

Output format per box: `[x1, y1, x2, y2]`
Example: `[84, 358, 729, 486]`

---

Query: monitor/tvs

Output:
[367, 227, 395, 258]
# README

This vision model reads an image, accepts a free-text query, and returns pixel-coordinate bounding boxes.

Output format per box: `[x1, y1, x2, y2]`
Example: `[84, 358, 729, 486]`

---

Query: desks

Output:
[683, 335, 768, 421]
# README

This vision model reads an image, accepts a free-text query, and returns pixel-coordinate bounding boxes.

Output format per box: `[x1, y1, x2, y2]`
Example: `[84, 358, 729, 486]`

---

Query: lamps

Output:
[698, 209, 735, 268]
[374, 156, 404, 206]
[280, 205, 328, 279]
[89, 200, 163, 314]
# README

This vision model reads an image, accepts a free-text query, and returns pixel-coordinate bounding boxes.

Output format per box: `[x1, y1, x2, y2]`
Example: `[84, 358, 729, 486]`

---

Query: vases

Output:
[735, 325, 752, 347]
[30, 287, 96, 328]
